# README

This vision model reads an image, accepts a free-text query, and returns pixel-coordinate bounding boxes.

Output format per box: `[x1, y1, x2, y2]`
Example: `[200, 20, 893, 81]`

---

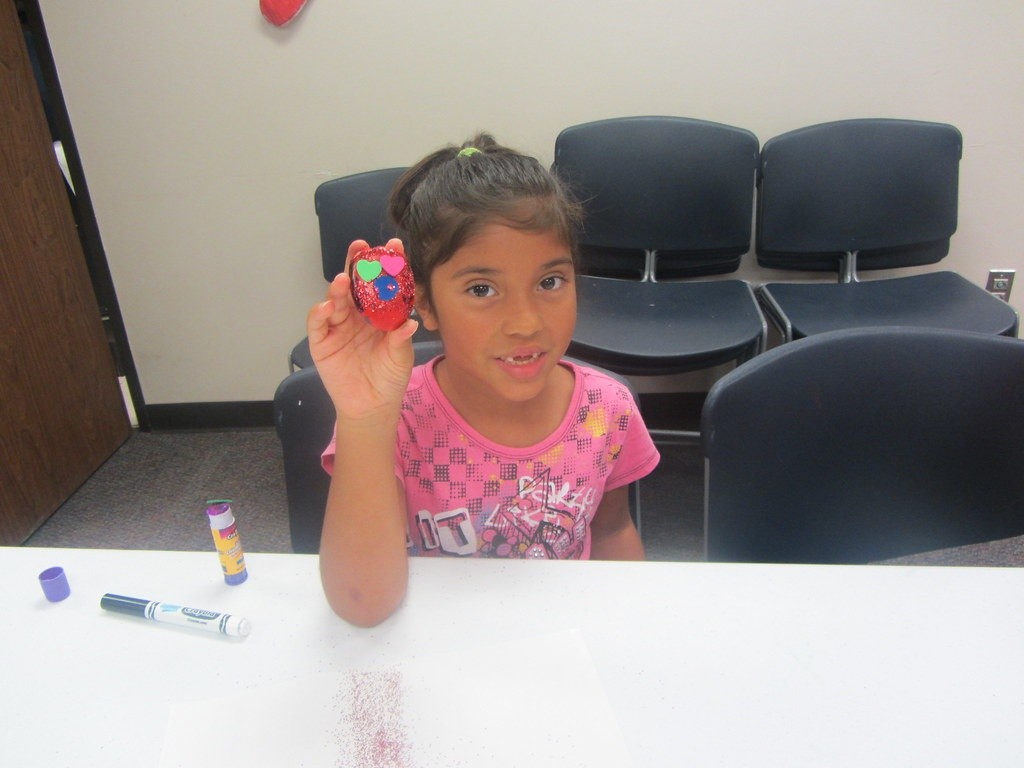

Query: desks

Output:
[0, 543, 1024, 768]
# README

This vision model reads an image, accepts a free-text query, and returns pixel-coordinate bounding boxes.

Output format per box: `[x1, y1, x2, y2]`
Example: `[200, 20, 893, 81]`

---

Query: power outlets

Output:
[985, 269, 1016, 304]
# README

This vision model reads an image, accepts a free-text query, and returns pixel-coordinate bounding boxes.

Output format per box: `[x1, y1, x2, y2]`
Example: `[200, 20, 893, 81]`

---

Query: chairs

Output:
[284, 166, 445, 373]
[274, 115, 1024, 569]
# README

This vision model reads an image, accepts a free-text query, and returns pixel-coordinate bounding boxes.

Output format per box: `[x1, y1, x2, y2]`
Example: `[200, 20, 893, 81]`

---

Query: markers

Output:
[101, 594, 249, 638]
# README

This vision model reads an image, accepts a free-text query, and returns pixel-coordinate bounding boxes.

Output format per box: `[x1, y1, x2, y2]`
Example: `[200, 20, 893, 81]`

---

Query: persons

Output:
[308, 133, 660, 625]
